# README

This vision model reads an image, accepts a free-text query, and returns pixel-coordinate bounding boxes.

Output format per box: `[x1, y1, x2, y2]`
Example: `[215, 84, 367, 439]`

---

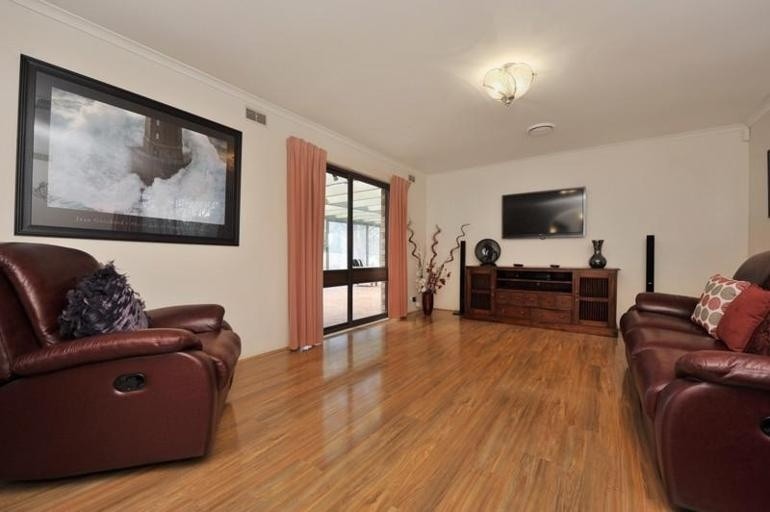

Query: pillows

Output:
[672, 272, 770, 390]
[52, 260, 149, 338]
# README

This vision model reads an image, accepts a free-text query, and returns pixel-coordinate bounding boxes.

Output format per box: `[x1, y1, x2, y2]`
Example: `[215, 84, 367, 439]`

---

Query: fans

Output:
[474, 239, 502, 267]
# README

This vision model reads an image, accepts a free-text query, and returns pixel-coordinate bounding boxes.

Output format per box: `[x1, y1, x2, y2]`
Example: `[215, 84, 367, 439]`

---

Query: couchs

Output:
[620, 250, 770, 512]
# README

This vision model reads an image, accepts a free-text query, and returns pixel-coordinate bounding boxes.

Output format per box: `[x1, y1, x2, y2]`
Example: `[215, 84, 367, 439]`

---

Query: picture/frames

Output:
[14, 53, 243, 247]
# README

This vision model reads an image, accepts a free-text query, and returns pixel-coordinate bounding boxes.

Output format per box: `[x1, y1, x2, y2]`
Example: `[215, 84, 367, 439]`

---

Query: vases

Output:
[421, 290, 434, 316]
[589, 240, 607, 270]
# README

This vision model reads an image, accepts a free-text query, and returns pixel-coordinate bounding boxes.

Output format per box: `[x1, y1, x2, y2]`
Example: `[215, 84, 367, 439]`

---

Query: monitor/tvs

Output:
[501, 186, 585, 239]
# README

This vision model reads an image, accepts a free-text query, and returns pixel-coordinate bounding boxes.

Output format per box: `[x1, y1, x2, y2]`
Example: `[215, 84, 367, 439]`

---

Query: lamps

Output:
[483, 63, 535, 112]
[525, 122, 555, 138]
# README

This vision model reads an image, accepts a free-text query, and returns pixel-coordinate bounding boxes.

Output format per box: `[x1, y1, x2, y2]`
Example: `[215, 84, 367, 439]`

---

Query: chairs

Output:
[0, 240, 241, 483]
[350, 258, 378, 287]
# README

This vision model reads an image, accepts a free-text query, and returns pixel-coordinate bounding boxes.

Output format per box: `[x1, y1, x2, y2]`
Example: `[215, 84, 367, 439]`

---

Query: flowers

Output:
[414, 262, 452, 295]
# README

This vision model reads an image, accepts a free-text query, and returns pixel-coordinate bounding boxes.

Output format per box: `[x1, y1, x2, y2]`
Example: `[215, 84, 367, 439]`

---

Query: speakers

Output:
[460, 241, 466, 313]
[646, 235, 654, 292]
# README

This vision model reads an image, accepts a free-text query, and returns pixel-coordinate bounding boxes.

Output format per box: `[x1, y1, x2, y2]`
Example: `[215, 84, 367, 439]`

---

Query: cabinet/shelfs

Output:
[463, 265, 621, 337]
